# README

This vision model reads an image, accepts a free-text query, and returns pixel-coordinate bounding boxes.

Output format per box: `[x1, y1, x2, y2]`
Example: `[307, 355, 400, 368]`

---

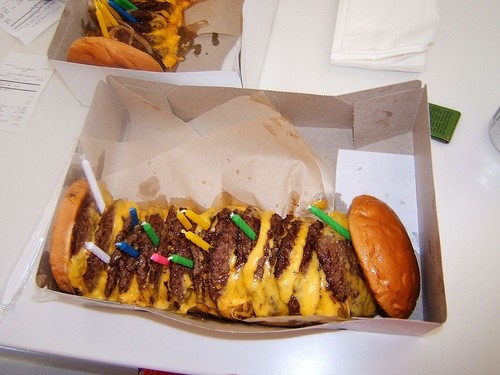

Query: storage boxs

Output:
[35, 74, 448, 334]
[46, 0, 279, 108]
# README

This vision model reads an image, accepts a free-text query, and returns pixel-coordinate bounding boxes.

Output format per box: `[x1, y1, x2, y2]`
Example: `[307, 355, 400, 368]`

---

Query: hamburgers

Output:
[48, 176, 421, 321]
[65, 0, 208, 74]
